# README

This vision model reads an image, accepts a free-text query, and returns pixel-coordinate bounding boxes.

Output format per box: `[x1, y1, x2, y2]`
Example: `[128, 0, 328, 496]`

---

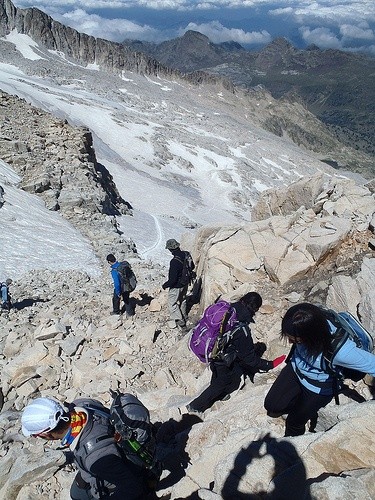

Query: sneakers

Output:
[181, 405, 198, 414]
[110, 312, 119, 315]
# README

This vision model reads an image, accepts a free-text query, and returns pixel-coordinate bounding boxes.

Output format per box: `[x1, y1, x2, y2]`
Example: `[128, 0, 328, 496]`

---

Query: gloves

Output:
[273, 354, 286, 367]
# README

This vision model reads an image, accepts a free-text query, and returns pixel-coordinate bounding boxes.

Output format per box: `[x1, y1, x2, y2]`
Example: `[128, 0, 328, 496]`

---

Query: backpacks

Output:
[187, 300, 247, 367]
[75, 389, 157, 478]
[0, 282, 6, 298]
[174, 251, 196, 285]
[323, 309, 375, 382]
[113, 261, 137, 295]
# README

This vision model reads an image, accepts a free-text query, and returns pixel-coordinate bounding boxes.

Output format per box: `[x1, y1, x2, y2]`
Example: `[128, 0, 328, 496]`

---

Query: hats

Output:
[20, 398, 63, 438]
[165, 239, 180, 249]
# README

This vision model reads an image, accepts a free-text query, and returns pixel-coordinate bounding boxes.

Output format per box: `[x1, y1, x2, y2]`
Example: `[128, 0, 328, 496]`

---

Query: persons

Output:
[160, 238, 197, 329]
[186, 291, 286, 414]
[0, 278, 13, 309]
[105, 253, 138, 316]
[263, 303, 375, 437]
[22, 393, 153, 500]
